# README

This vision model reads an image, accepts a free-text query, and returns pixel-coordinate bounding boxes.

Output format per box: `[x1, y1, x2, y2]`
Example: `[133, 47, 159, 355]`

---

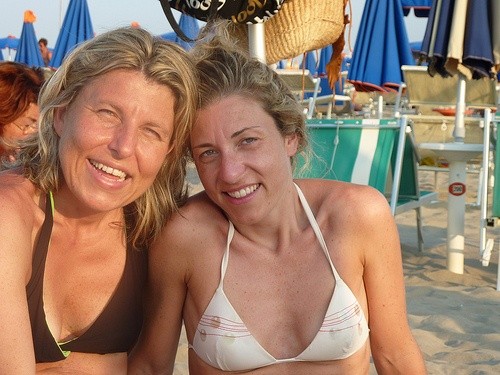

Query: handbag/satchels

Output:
[227, 0, 344, 66]
[159, 0, 285, 43]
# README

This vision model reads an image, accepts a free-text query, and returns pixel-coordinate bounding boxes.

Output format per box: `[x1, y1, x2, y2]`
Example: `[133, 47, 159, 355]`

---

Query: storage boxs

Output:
[401, 65, 496, 159]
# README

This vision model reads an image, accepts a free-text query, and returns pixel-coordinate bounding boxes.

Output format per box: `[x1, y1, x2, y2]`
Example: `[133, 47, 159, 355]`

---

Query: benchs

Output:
[480, 107, 500, 266]
[292, 118, 436, 255]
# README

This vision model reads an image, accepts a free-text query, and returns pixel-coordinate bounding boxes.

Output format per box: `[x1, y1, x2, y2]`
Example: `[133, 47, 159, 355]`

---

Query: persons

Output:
[38, 38, 52, 67]
[127, 33, 426, 374]
[0, 61, 47, 169]
[0, 25, 197, 375]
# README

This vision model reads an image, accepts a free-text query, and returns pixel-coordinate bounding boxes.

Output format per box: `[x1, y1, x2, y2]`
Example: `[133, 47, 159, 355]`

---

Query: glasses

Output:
[12, 120, 34, 136]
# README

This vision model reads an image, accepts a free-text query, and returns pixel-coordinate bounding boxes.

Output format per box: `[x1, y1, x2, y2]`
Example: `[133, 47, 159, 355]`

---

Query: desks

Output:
[419, 141, 483, 275]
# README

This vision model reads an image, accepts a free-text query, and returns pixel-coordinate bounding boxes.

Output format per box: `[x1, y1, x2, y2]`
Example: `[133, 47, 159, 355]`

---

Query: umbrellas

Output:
[317, 44, 349, 96]
[419, 0, 500, 276]
[14, 10, 47, 70]
[173, 10, 201, 48]
[298, 50, 320, 100]
[345, 0, 418, 98]
[48, 0, 97, 69]
